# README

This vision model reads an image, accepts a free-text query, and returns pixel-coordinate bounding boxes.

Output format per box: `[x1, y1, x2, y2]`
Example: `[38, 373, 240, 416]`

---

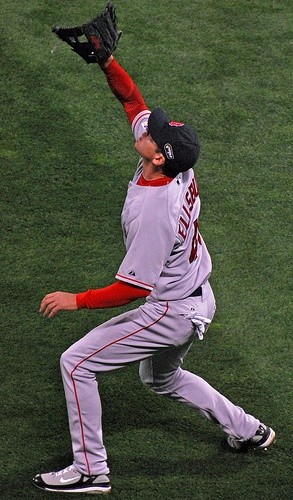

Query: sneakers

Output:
[227, 422, 277, 451]
[32, 464, 111, 494]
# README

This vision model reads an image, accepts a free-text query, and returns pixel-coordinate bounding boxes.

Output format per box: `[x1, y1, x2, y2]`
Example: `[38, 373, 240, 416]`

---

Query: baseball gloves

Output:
[49, 0, 122, 66]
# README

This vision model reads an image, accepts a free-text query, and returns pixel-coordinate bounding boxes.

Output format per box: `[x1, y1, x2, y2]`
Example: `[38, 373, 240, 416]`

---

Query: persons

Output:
[30, 3, 276, 494]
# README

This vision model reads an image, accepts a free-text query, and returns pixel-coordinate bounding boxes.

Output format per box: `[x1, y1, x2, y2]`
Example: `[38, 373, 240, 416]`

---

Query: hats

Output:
[147, 107, 198, 179]
